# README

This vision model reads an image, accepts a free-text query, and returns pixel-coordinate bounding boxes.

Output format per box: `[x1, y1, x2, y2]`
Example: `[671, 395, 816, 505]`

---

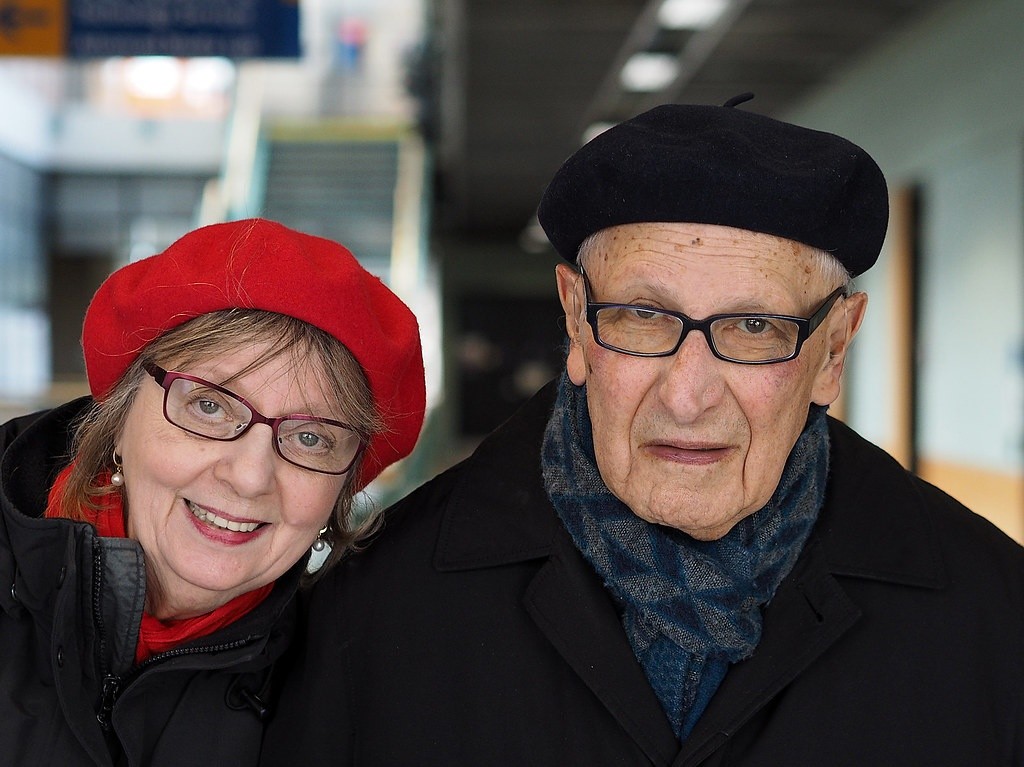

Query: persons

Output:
[251, 104, 1024, 767]
[1, 216, 427, 767]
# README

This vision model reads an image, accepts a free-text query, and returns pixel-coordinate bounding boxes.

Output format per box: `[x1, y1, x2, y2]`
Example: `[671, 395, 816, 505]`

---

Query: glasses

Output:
[579, 265, 849, 366]
[139, 353, 371, 476]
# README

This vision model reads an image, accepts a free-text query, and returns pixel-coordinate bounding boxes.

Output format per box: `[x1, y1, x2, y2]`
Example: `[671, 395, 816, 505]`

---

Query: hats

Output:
[538, 93, 890, 279]
[83, 216, 428, 500]
